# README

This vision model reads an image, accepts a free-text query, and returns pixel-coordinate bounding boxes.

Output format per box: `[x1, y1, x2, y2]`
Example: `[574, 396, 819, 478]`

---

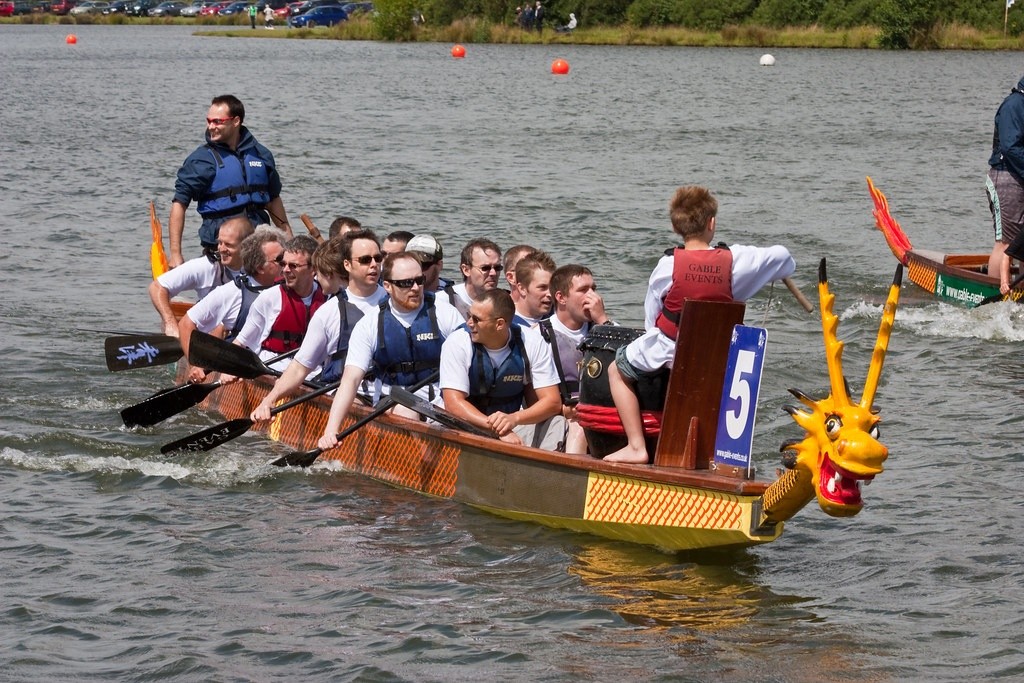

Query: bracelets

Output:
[603, 321, 611, 325]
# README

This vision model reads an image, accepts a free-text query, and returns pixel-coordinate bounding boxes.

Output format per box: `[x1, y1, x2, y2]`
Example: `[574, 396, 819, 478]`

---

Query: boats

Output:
[866, 176, 1024, 308]
[148, 200, 903, 553]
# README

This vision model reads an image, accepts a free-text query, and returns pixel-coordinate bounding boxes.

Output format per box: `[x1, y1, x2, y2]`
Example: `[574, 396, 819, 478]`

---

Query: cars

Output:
[291, 6, 348, 28]
[0, 0, 373, 17]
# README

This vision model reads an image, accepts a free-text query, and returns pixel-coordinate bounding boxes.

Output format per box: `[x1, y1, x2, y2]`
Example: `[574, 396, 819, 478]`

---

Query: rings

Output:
[193, 379, 197, 382]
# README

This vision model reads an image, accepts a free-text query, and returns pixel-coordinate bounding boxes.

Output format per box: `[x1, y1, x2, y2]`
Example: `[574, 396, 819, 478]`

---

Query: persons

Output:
[250, 229, 388, 425]
[512, 253, 556, 329]
[567, 13, 577, 33]
[404, 233, 452, 294]
[283, 2, 292, 28]
[502, 245, 535, 305]
[317, 252, 467, 452]
[534, 1, 543, 31]
[515, 7, 524, 30]
[169, 95, 294, 271]
[149, 217, 363, 388]
[605, 187, 796, 463]
[440, 288, 569, 452]
[264, 4, 274, 28]
[533, 264, 620, 455]
[381, 231, 414, 266]
[413, 14, 425, 26]
[987, 75, 1024, 293]
[523, 4, 535, 30]
[436, 238, 504, 322]
[248, 4, 257, 29]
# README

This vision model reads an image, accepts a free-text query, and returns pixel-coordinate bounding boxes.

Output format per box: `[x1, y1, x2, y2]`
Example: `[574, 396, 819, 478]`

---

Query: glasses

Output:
[264, 254, 282, 264]
[469, 265, 503, 272]
[466, 312, 503, 324]
[278, 261, 308, 269]
[206, 117, 235, 125]
[385, 275, 427, 287]
[419, 260, 437, 272]
[354, 254, 383, 264]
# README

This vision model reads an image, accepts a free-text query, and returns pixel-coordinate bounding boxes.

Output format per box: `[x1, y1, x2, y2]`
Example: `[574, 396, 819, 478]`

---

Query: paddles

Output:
[187, 329, 324, 389]
[120, 347, 300, 429]
[390, 385, 498, 439]
[105, 332, 234, 372]
[974, 272, 1024, 308]
[142, 368, 214, 401]
[160, 365, 374, 458]
[269, 369, 440, 468]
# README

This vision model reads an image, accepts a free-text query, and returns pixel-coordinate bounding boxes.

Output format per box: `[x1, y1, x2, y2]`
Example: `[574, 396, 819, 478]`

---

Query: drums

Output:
[578, 325, 671, 463]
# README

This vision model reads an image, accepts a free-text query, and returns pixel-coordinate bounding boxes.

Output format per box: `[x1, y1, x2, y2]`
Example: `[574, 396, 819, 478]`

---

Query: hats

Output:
[404, 235, 442, 256]
